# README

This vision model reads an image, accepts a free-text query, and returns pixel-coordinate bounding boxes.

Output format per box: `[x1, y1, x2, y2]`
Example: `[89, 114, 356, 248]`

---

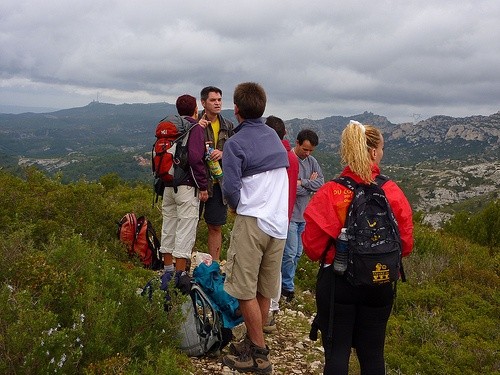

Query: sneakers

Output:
[228, 334, 270, 358]
[222, 341, 273, 375]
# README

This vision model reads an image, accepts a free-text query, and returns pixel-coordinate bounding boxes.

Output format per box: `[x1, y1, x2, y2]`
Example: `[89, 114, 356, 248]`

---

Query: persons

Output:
[281, 130, 324, 302]
[265, 115, 299, 314]
[222, 82, 289, 372]
[301, 120, 413, 375]
[159, 95, 208, 278]
[198, 86, 234, 263]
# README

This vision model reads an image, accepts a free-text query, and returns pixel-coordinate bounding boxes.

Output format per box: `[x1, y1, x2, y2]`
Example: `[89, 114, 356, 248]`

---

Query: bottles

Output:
[205, 144, 224, 178]
[334, 227, 348, 272]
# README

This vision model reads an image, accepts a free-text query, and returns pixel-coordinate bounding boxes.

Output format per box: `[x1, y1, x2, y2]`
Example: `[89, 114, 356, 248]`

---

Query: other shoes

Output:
[262, 317, 276, 332]
[281, 288, 295, 299]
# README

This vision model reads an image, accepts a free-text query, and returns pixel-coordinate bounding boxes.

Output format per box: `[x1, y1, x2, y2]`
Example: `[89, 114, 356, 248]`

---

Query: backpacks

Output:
[191, 260, 245, 328]
[141, 269, 192, 313]
[331, 175, 401, 285]
[167, 282, 223, 357]
[152, 114, 195, 183]
[120, 213, 162, 271]
